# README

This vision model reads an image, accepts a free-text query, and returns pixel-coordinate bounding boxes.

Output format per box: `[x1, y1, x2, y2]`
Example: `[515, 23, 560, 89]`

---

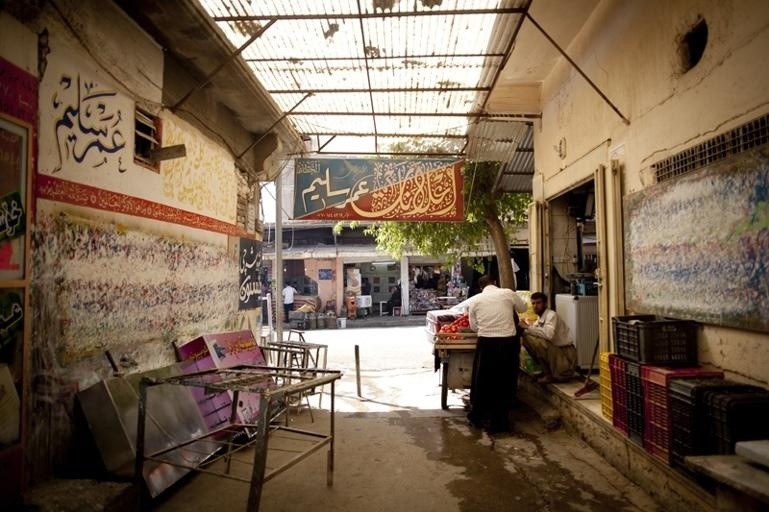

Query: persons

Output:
[388, 286, 401, 314]
[516, 295, 579, 386]
[468, 274, 527, 433]
[281, 281, 297, 322]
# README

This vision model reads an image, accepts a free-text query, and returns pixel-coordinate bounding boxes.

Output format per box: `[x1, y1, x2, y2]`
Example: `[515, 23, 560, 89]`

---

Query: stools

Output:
[392, 306, 401, 317]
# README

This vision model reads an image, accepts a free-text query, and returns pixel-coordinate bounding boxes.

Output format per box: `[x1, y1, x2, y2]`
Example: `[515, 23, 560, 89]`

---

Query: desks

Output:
[379, 301, 390, 317]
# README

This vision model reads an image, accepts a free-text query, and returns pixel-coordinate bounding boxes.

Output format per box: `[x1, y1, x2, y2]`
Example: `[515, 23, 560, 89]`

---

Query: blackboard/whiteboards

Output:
[623, 145, 769, 335]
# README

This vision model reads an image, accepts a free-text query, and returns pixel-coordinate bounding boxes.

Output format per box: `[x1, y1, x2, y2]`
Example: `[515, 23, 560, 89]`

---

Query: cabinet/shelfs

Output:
[409, 288, 440, 315]
[130, 329, 343, 512]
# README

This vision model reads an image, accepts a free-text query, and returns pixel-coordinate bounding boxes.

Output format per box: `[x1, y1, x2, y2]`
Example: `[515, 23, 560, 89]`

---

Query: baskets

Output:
[597, 352, 769, 471]
[611, 313, 705, 369]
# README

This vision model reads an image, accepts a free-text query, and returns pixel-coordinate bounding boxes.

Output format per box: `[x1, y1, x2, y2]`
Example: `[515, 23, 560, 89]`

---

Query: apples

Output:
[438, 315, 470, 340]
